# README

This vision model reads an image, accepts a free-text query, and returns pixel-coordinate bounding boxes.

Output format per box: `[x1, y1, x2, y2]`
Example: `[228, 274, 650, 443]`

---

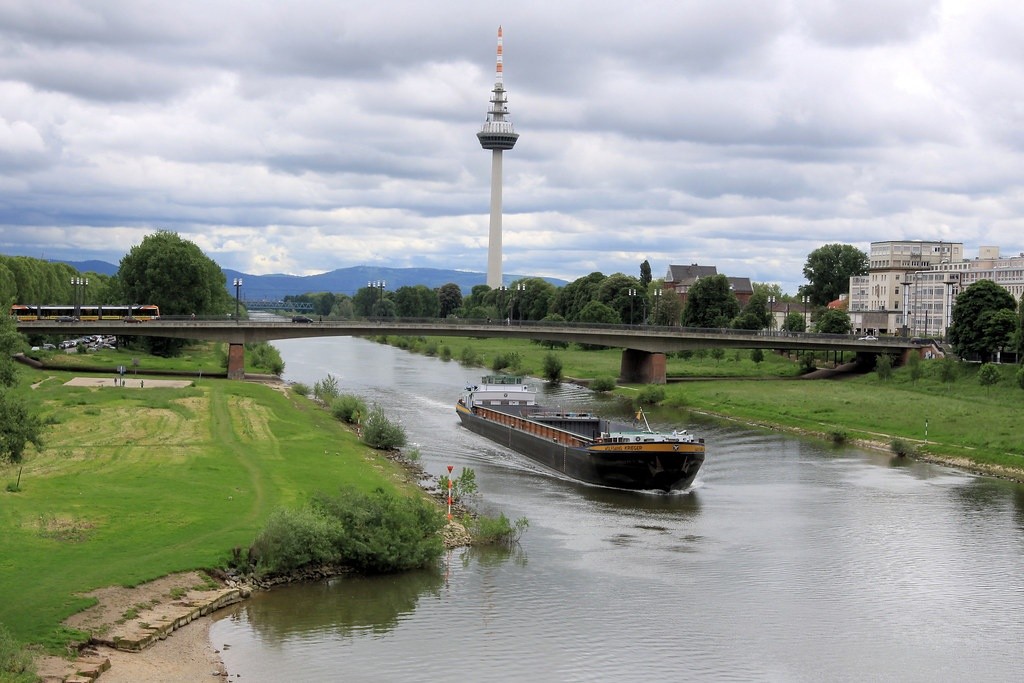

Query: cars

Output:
[292, 314, 313, 323]
[55, 317, 80, 322]
[123, 317, 142, 323]
[858, 335, 879, 341]
[31, 334, 116, 350]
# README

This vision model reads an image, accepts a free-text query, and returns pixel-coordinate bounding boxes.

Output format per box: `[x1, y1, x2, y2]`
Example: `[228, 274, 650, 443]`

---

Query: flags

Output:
[632, 410, 640, 428]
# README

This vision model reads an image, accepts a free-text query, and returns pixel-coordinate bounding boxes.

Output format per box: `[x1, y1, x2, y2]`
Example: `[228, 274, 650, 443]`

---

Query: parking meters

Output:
[367, 280, 376, 321]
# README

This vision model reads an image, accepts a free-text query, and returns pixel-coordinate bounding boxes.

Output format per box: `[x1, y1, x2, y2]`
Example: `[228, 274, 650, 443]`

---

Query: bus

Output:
[9, 305, 161, 320]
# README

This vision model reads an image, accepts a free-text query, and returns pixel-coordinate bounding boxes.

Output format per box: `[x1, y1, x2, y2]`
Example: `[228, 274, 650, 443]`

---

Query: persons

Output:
[505, 317, 511, 325]
[319, 316, 322, 324]
[191, 313, 195, 320]
[487, 316, 490, 324]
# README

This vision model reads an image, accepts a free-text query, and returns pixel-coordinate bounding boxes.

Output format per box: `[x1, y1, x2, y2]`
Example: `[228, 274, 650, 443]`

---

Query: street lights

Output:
[768, 295, 775, 336]
[629, 288, 637, 329]
[801, 295, 810, 332]
[378, 280, 386, 324]
[500, 283, 508, 326]
[233, 279, 243, 323]
[654, 288, 662, 326]
[517, 283, 526, 328]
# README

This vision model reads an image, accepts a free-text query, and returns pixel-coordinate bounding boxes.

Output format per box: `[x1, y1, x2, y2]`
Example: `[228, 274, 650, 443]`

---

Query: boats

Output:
[455, 382, 705, 494]
